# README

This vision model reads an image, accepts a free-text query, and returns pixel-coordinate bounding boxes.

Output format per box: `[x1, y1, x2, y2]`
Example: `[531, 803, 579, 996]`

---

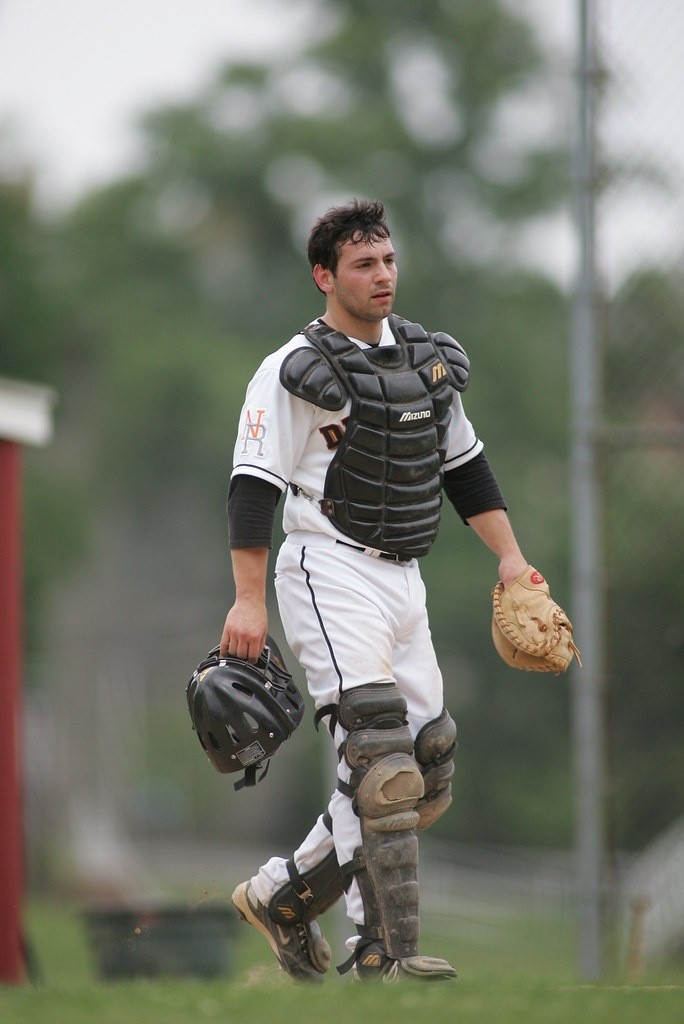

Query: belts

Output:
[335, 539, 412, 563]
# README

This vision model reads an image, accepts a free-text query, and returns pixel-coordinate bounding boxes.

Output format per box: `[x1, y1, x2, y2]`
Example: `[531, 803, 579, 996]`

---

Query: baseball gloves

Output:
[490, 562, 585, 674]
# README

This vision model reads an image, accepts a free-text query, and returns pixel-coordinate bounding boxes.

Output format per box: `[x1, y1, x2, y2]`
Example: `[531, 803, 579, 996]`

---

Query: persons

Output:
[219, 198, 552, 989]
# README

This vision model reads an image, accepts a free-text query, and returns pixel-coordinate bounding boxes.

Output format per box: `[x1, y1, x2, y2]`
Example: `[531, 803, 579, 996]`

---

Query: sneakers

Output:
[348, 960, 459, 989]
[231, 881, 325, 987]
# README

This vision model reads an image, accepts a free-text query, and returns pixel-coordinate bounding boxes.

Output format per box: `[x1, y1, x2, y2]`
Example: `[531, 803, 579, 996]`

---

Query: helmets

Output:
[186, 629, 304, 791]
